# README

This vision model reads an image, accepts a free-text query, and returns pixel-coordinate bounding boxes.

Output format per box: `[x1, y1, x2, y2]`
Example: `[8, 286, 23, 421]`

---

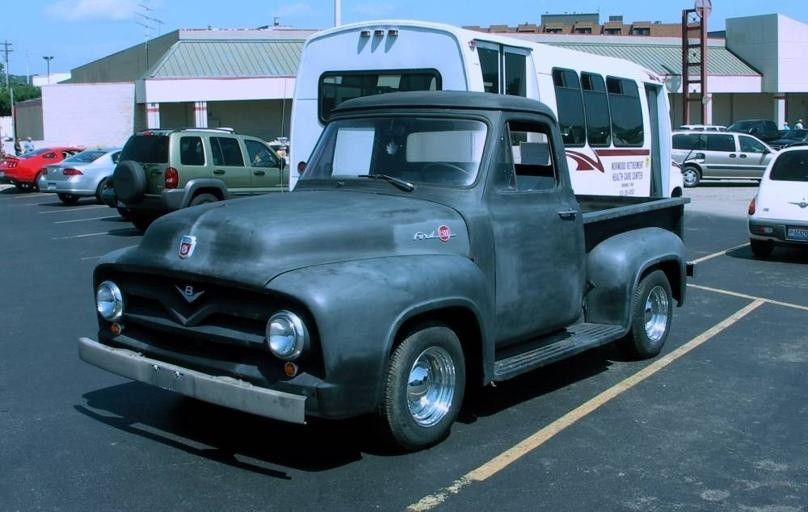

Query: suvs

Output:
[112, 128, 289, 218]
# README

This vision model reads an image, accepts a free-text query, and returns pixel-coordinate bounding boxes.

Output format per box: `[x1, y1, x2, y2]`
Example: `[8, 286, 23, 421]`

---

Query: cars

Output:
[746, 145, 808, 256]
[673, 119, 808, 187]
[0, 145, 122, 204]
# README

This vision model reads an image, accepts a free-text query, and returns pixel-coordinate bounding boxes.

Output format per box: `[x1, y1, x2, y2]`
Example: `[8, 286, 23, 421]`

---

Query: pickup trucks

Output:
[78, 90, 696, 449]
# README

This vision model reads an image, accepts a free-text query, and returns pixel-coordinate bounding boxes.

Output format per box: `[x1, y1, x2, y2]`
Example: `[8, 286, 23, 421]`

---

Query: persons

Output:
[795, 119, 804, 131]
[14, 136, 34, 156]
[783, 122, 791, 130]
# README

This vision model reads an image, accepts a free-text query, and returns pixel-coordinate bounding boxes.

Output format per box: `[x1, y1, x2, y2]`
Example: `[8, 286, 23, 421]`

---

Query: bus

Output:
[289, 20, 683, 196]
